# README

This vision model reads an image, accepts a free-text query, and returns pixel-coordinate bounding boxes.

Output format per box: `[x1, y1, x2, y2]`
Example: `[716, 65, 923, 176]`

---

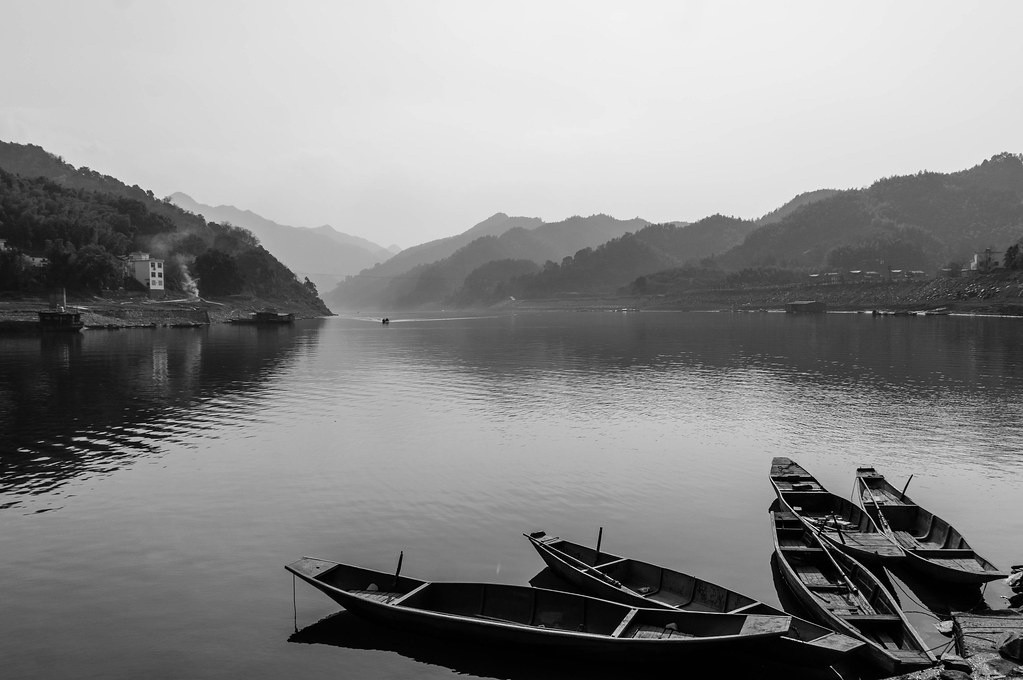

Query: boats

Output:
[852, 463, 1009, 585]
[229, 308, 296, 323]
[37, 306, 85, 333]
[382, 318, 389, 322]
[768, 497, 938, 671]
[770, 455, 907, 562]
[283, 555, 793, 657]
[523, 530, 868, 655]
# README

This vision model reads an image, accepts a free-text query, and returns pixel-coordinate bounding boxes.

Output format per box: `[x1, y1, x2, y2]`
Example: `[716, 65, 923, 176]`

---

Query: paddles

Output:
[522, 533, 642, 596]
[811, 531, 876, 615]
[860, 476, 897, 543]
[802, 516, 837, 531]
